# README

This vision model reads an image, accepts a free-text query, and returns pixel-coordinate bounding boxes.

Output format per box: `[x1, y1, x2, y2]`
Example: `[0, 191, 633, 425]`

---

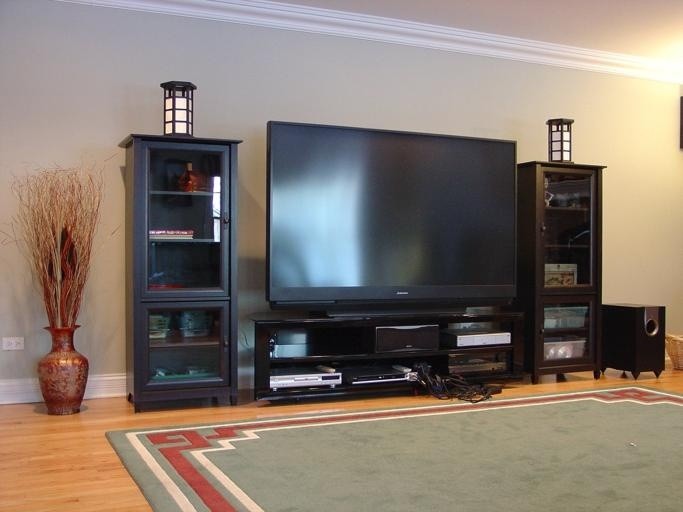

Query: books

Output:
[148, 228, 194, 240]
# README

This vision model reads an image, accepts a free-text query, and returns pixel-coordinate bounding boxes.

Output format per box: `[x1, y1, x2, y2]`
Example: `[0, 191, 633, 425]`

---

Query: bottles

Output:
[177, 161, 201, 192]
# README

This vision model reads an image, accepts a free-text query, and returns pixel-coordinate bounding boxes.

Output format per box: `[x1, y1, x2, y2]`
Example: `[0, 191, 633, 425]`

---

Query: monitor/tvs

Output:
[266, 120, 517, 317]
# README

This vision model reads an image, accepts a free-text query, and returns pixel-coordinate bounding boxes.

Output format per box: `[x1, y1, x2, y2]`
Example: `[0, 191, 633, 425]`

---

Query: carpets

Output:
[100, 385, 682, 512]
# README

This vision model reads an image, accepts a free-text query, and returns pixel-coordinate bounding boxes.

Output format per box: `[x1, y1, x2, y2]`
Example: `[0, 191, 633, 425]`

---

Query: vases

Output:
[33, 321, 92, 418]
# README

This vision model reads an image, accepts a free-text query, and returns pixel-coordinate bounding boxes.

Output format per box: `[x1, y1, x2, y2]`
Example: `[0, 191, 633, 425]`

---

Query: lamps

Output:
[547, 117, 577, 166]
[157, 78, 197, 134]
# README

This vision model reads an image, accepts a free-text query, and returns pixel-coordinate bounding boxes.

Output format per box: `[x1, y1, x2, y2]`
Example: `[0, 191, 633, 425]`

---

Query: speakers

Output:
[374, 322, 441, 354]
[602, 303, 666, 380]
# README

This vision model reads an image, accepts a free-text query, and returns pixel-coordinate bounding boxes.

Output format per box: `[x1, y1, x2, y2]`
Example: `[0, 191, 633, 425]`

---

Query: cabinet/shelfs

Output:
[248, 302, 527, 407]
[116, 132, 246, 414]
[514, 156, 606, 384]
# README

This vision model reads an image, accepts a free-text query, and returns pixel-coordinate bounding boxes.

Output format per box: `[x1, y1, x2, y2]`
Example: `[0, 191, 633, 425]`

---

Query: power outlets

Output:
[1, 335, 27, 353]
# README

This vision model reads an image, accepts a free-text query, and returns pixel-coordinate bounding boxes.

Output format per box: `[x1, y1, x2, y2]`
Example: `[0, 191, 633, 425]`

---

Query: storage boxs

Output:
[542, 261, 580, 289]
[273, 328, 309, 344]
[540, 337, 587, 362]
[538, 305, 590, 331]
[271, 341, 312, 360]
[145, 309, 215, 340]
